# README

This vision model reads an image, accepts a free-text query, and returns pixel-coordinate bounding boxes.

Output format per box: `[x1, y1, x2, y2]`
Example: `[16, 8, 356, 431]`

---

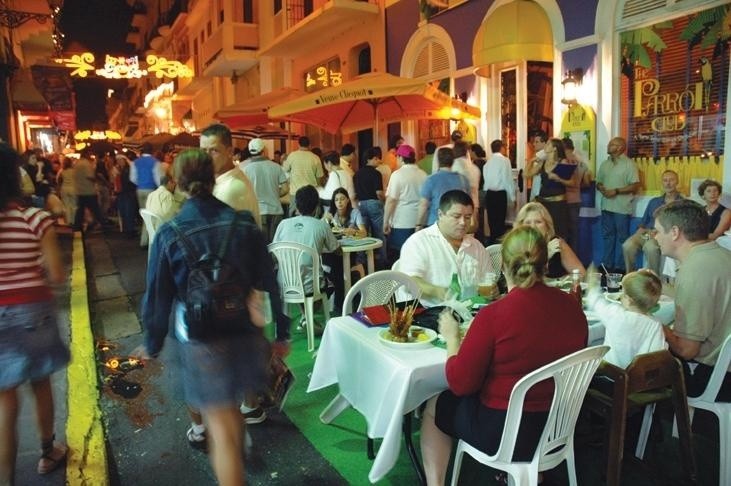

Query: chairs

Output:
[138, 207, 166, 270]
[320, 255, 366, 311]
[319, 270, 422, 424]
[634, 334, 730, 486]
[266, 241, 330, 352]
[551, 350, 698, 485]
[391, 259, 402, 302]
[484, 244, 504, 284]
[450, 345, 611, 485]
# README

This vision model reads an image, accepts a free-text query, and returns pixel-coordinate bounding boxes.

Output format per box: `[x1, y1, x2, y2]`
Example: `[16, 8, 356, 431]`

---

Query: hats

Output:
[393, 145, 415, 159]
[248, 138, 265, 154]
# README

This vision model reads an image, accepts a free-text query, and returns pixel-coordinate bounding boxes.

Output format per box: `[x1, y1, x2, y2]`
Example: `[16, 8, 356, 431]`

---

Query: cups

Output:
[478, 283, 494, 295]
[607, 271, 622, 293]
[584, 273, 603, 294]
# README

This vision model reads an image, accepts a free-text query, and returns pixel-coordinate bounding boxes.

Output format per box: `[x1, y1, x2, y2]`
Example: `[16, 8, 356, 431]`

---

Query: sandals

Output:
[38, 435, 66, 474]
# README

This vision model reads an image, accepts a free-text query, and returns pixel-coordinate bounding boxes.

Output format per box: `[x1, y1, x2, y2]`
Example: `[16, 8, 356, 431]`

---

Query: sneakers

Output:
[240, 404, 266, 424]
[187, 428, 208, 452]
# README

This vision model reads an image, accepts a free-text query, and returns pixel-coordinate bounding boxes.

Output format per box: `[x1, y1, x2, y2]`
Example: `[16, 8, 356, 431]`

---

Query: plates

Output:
[331, 228, 344, 233]
[379, 325, 439, 346]
[546, 280, 588, 292]
[604, 292, 626, 304]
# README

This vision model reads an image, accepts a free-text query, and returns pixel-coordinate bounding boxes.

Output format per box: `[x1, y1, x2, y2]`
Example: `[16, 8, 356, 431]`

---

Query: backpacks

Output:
[166, 212, 245, 342]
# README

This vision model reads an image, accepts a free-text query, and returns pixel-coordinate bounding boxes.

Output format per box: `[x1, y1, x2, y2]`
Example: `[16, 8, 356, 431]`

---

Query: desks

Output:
[319, 236, 383, 315]
[328, 281, 677, 486]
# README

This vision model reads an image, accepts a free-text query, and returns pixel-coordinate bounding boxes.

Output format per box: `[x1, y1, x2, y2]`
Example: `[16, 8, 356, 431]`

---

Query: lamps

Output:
[558, 66, 587, 123]
[447, 89, 469, 128]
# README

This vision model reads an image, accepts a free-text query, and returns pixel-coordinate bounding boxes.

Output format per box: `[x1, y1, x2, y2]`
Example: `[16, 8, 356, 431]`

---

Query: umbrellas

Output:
[214, 84, 309, 153]
[269, 69, 481, 147]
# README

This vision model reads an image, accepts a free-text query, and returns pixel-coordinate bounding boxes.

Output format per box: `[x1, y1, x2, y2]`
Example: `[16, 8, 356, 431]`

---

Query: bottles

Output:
[568, 268, 584, 306]
[450, 271, 462, 302]
